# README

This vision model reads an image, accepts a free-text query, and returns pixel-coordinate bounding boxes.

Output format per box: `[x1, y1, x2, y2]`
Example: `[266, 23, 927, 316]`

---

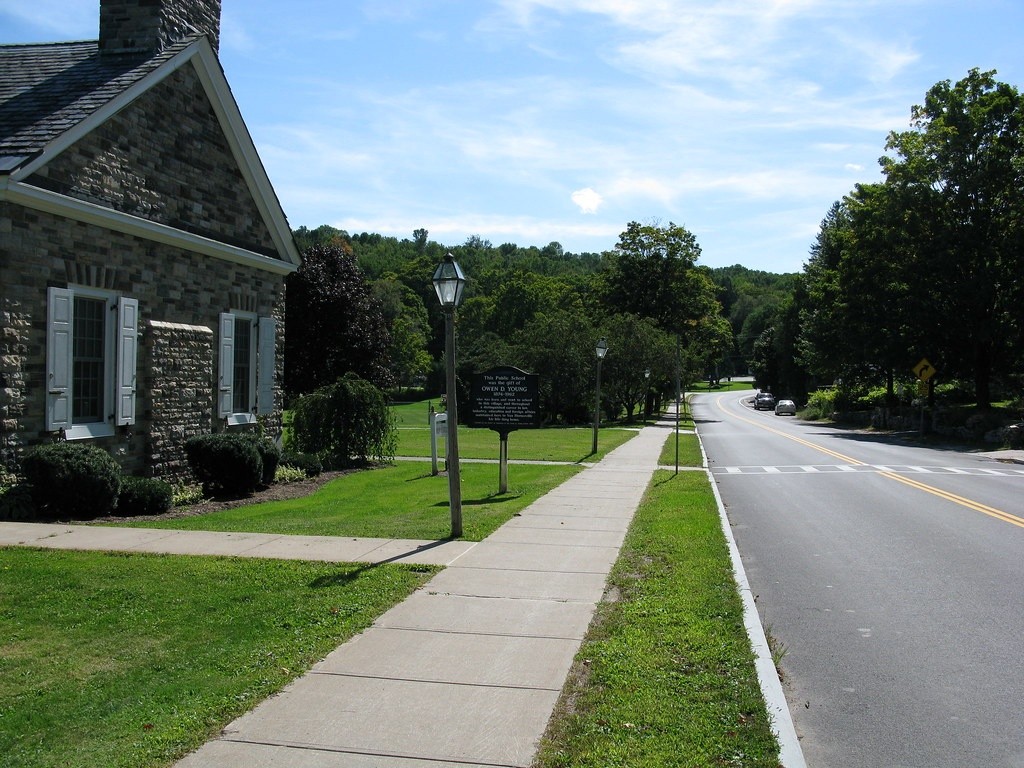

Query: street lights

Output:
[431, 249, 469, 538]
[642, 368, 651, 423]
[592, 336, 609, 454]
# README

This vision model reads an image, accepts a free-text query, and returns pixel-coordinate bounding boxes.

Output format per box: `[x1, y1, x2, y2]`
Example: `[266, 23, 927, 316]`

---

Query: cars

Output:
[774, 399, 796, 416]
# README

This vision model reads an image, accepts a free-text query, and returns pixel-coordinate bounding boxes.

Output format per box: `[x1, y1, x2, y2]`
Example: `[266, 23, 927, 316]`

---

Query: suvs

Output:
[754, 392, 775, 410]
